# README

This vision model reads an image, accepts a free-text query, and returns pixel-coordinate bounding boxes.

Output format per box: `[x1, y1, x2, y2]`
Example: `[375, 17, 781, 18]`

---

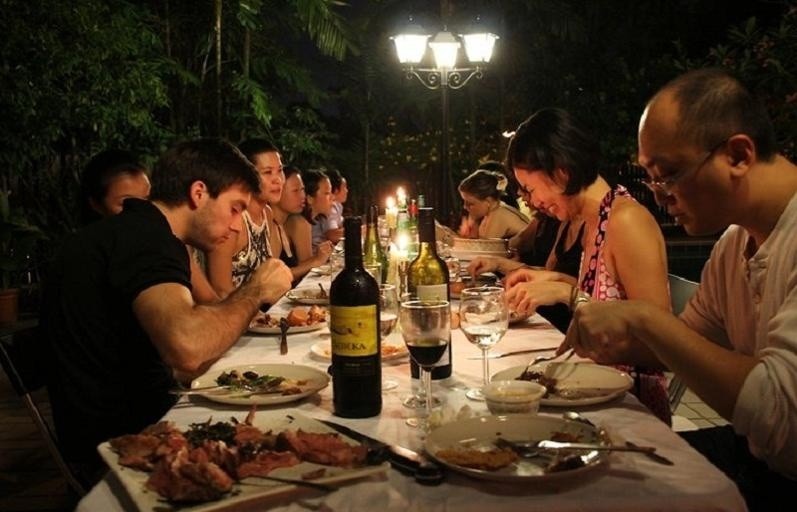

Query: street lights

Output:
[386, 15, 500, 226]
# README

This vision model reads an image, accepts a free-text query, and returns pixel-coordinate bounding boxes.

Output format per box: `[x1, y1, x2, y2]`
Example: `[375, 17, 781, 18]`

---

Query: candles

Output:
[384, 195, 397, 230]
[388, 237, 408, 285]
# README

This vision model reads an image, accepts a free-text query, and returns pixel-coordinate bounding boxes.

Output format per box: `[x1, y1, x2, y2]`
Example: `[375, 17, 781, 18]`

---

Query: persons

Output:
[456, 157, 587, 333]
[40, 136, 295, 504]
[501, 107, 674, 432]
[204, 138, 287, 320]
[268, 164, 352, 284]
[557, 66, 796, 511]
[69, 146, 223, 390]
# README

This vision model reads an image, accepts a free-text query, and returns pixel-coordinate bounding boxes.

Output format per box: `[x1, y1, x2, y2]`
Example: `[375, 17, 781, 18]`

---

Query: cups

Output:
[481, 381, 544, 415]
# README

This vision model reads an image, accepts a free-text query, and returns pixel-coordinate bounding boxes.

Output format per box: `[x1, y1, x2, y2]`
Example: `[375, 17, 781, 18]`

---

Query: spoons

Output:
[563, 411, 674, 466]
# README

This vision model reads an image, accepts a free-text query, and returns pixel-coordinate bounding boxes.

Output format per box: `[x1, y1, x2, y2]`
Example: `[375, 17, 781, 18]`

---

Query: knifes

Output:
[467, 346, 560, 360]
[287, 413, 444, 486]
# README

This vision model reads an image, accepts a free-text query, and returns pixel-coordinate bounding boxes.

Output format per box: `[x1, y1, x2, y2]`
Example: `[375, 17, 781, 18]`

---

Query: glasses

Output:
[640, 141, 727, 196]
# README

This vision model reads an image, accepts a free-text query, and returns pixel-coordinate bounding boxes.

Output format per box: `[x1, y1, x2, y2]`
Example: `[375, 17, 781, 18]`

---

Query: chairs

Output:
[666, 273, 699, 416]
[0, 325, 86, 497]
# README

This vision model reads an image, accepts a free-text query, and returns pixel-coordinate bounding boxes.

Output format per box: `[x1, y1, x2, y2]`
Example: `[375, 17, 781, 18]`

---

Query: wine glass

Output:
[459, 286, 510, 402]
[245, 194, 457, 429]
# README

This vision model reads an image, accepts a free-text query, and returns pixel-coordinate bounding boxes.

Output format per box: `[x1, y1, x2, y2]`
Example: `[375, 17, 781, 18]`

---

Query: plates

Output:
[423, 416, 613, 481]
[492, 362, 636, 407]
[98, 409, 389, 512]
[192, 363, 329, 406]
